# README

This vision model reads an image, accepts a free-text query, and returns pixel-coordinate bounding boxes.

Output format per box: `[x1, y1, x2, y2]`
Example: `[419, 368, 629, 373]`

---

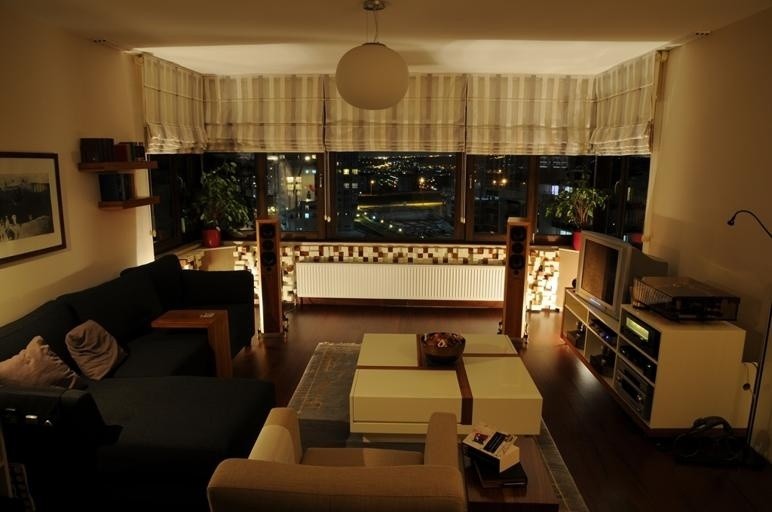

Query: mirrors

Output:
[741, 310, 772, 472]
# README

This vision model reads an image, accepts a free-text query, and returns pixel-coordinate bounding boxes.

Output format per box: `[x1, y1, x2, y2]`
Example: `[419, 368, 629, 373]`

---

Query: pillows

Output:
[65, 319, 128, 379]
[0, 331, 89, 392]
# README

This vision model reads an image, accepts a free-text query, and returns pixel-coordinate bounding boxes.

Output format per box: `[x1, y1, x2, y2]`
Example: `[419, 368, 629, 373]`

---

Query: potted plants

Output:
[185, 158, 253, 249]
[543, 184, 610, 253]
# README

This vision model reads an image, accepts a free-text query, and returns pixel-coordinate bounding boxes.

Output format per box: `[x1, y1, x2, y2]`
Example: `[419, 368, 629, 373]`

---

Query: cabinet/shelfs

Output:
[78, 158, 163, 210]
[558, 286, 746, 429]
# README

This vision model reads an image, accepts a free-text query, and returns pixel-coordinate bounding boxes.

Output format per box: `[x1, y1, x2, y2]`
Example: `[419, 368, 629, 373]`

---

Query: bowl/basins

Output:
[422, 331, 466, 363]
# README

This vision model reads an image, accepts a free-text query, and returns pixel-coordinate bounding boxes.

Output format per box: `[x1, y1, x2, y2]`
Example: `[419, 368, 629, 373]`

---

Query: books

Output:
[461, 421, 520, 472]
[471, 456, 528, 489]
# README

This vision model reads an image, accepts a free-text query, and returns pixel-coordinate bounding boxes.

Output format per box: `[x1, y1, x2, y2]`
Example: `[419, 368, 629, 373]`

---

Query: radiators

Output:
[295, 260, 504, 302]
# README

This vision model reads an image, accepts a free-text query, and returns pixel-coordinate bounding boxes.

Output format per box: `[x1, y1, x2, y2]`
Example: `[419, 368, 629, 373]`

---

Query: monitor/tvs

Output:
[575, 229, 667, 321]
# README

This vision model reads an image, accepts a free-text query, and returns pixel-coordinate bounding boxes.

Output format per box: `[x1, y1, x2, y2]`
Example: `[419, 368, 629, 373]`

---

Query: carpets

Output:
[287, 339, 589, 512]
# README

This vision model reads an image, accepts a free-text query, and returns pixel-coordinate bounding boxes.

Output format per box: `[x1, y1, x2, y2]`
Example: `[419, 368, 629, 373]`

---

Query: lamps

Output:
[726, 207, 772, 238]
[334, 0, 410, 112]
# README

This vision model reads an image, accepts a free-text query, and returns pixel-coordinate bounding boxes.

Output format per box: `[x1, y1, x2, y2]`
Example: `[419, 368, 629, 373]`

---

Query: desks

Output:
[151, 306, 237, 382]
[467, 435, 560, 510]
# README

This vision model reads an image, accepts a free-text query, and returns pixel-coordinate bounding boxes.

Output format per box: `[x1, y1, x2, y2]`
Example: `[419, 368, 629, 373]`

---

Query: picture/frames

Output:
[1, 150, 67, 266]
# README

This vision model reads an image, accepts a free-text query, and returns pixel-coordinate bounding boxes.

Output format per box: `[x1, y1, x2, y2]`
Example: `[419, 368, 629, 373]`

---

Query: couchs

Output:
[0, 254, 255, 489]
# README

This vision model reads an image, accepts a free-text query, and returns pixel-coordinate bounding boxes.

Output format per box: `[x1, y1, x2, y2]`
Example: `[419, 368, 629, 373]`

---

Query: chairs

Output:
[206, 398, 472, 510]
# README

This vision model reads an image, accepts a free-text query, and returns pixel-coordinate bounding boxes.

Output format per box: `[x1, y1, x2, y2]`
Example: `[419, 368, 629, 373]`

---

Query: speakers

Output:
[500, 216, 531, 349]
[255, 217, 287, 346]
